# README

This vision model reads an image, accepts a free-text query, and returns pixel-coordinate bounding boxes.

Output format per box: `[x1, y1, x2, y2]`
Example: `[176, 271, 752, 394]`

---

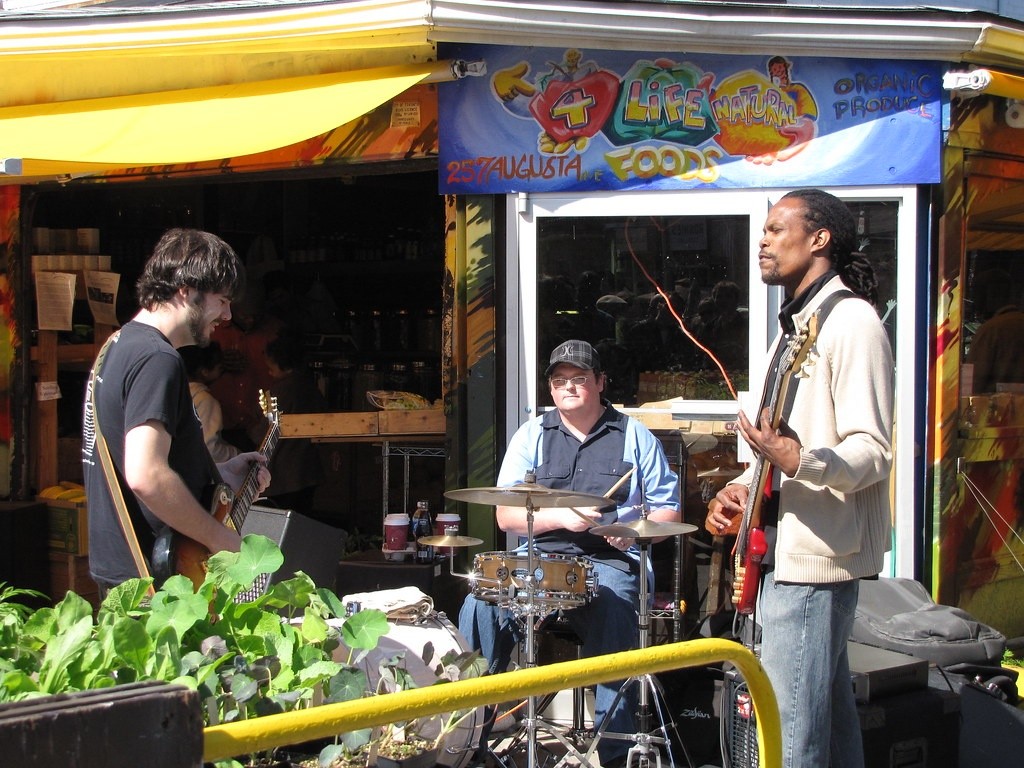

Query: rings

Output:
[620, 541, 625, 546]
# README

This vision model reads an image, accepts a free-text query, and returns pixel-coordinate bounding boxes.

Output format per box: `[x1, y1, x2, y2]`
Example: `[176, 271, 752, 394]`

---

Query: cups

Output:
[435, 514, 461, 556]
[385, 513, 410, 550]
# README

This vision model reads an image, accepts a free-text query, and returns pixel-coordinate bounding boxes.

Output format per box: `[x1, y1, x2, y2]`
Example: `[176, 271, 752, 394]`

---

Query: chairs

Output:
[645, 429, 684, 619]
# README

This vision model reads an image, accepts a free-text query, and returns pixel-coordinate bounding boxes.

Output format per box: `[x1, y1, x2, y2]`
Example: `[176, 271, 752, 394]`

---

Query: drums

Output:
[470, 552, 593, 611]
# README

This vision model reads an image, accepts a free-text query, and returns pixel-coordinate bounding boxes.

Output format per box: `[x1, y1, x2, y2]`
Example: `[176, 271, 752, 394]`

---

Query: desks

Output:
[331, 550, 467, 608]
[275, 408, 449, 534]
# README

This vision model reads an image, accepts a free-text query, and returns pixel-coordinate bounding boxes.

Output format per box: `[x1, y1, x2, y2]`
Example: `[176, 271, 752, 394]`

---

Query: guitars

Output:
[727, 312, 819, 615]
[153, 386, 279, 621]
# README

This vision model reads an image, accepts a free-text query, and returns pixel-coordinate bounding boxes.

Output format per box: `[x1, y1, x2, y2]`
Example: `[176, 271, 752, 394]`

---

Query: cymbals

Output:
[442, 483, 618, 510]
[596, 521, 701, 541]
[420, 532, 489, 547]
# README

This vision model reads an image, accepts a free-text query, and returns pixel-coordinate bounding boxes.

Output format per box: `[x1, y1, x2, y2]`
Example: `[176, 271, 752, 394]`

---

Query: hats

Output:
[543, 340, 601, 381]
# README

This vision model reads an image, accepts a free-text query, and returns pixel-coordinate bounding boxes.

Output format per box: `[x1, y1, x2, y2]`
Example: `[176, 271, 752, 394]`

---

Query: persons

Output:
[538, 270, 747, 394]
[458, 340, 686, 766]
[79, 228, 329, 604]
[705, 186, 897, 768]
[970, 267, 1024, 393]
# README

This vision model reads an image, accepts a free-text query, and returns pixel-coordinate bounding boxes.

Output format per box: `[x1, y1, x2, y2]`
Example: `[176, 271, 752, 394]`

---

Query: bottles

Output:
[413, 502, 432, 564]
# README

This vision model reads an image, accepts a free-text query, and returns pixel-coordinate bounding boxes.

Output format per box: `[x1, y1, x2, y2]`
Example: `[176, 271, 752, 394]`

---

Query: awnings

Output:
[1, 59, 433, 165]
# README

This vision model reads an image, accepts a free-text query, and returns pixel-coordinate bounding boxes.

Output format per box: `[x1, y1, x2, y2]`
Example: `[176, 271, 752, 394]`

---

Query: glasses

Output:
[551, 373, 595, 389]
